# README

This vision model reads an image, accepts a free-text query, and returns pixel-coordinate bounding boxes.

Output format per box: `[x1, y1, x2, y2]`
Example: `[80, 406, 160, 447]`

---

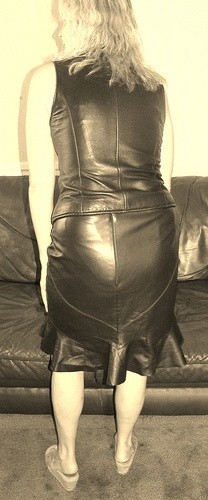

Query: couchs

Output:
[0, 176, 208, 416]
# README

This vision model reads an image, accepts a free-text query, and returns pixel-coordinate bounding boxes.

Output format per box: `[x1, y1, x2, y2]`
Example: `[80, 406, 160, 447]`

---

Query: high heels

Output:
[45, 444, 79, 492]
[114, 434, 138, 475]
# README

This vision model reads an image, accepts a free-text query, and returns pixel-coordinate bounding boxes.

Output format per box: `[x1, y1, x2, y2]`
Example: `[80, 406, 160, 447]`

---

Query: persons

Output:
[25, 0, 188, 491]
